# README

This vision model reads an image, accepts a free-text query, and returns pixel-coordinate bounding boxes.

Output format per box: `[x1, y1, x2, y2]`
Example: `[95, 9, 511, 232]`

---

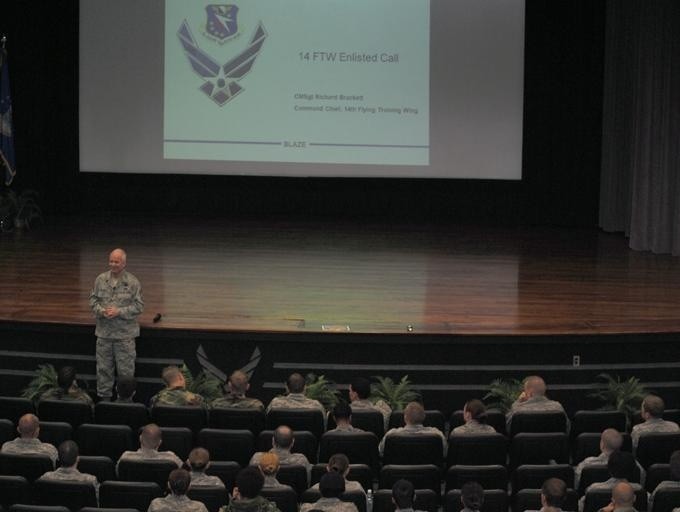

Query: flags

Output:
[0, 46, 16, 186]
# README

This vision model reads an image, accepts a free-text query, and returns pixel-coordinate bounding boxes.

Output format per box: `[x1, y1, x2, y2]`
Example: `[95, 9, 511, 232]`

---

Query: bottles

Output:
[367, 488, 373, 511]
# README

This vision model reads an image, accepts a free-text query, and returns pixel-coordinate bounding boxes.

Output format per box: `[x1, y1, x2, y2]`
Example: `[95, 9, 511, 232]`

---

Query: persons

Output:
[115, 364, 325, 417]
[41, 365, 94, 410]
[303, 454, 367, 511]
[539, 479, 567, 510]
[116, 424, 312, 512]
[323, 379, 392, 433]
[506, 375, 569, 436]
[2, 413, 58, 463]
[575, 395, 678, 511]
[392, 480, 485, 512]
[89, 248, 144, 401]
[378, 402, 448, 457]
[40, 440, 99, 504]
[451, 399, 497, 433]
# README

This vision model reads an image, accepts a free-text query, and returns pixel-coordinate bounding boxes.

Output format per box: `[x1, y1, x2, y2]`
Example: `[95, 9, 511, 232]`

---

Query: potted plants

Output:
[0, 188, 44, 234]
